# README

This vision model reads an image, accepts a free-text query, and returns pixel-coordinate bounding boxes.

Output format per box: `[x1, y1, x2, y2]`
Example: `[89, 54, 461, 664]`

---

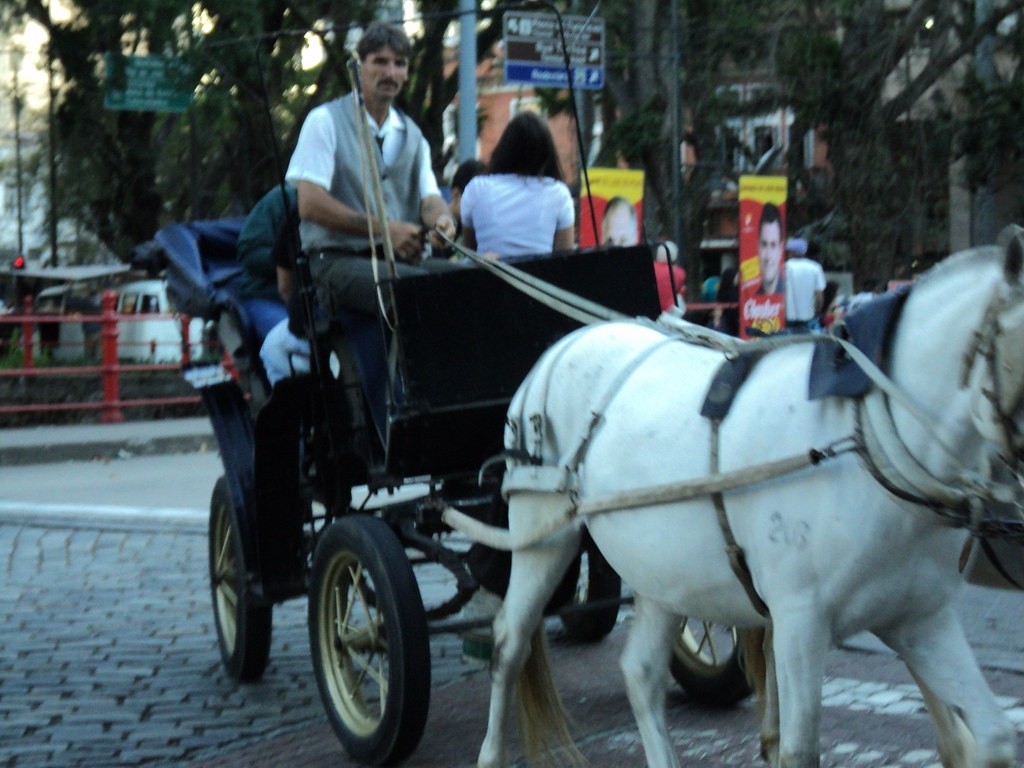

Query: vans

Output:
[20, 278, 206, 365]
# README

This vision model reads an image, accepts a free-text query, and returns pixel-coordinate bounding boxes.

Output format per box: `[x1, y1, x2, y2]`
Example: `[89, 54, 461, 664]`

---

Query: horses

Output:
[475, 226, 1024, 768]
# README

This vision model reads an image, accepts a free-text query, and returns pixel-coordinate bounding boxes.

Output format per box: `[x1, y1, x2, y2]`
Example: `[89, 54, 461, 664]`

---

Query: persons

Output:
[233, 180, 315, 336]
[682, 265, 739, 335]
[754, 203, 785, 295]
[653, 241, 686, 314]
[282, 23, 458, 322]
[582, 196, 638, 253]
[785, 238, 825, 333]
[431, 110, 580, 269]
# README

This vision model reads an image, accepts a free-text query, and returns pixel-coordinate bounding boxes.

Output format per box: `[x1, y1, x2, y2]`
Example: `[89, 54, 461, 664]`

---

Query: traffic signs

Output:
[504, 12, 607, 90]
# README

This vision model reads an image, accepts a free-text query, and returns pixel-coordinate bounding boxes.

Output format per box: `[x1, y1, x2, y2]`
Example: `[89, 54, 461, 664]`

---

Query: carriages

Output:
[207, 228, 1024, 768]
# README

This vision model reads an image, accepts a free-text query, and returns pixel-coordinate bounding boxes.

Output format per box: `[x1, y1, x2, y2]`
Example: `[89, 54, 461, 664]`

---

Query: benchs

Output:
[134, 214, 399, 443]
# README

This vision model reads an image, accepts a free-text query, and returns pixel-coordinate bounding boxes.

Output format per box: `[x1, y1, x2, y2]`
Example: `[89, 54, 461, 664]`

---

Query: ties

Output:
[375, 134, 384, 157]
